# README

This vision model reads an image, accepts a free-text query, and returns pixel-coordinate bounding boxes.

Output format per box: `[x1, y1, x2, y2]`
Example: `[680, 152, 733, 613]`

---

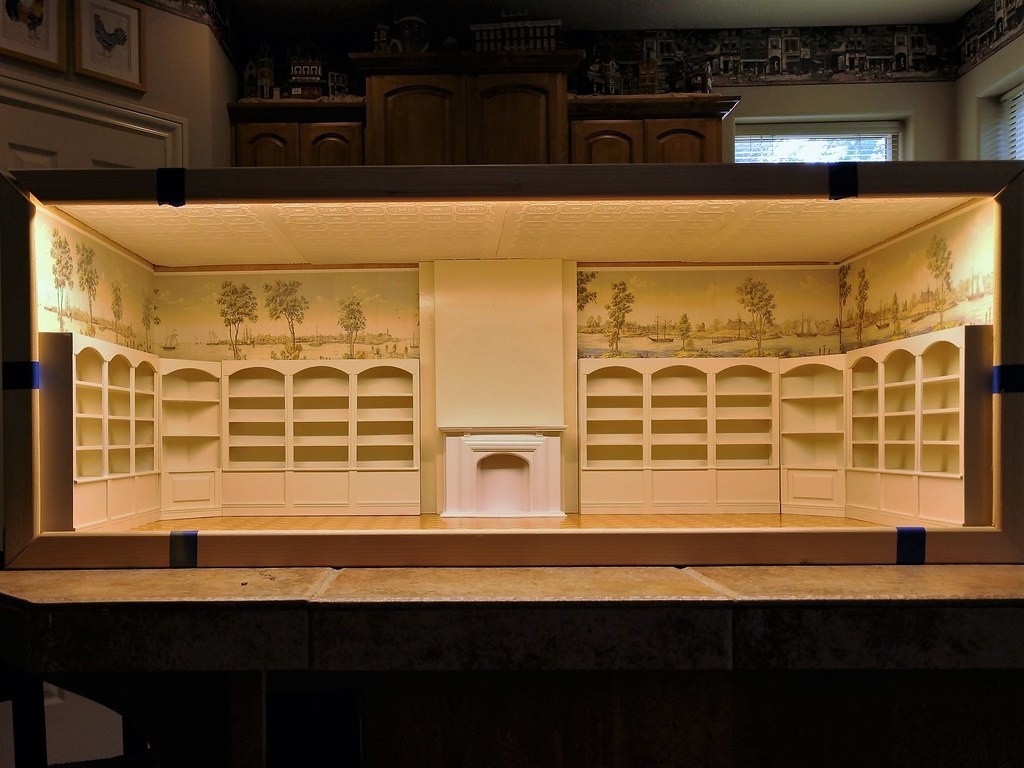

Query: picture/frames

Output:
[0, 0, 67, 75]
[73, 0, 148, 96]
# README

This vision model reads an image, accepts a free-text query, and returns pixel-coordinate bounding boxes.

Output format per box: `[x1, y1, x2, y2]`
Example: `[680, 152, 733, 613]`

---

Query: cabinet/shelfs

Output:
[39, 332, 161, 532]
[227, 101, 366, 165]
[779, 354, 845, 518]
[348, 49, 586, 165]
[158, 357, 222, 521]
[577, 357, 781, 515]
[567, 94, 742, 164]
[221, 359, 421, 517]
[845, 325, 993, 527]
